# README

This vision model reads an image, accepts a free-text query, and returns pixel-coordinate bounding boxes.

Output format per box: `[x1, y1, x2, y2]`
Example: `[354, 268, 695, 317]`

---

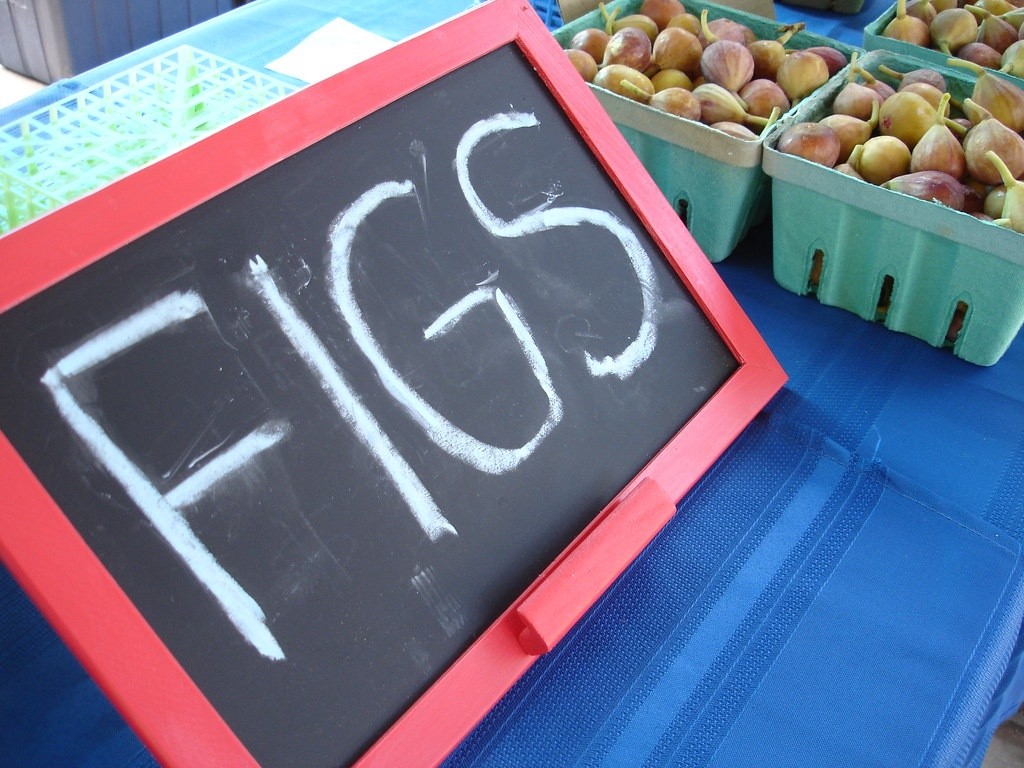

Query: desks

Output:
[1, 0, 1024, 767]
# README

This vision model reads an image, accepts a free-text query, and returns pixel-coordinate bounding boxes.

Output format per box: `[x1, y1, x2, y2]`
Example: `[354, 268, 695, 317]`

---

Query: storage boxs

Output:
[864, 0, 1024, 89]
[549, 0, 869, 262]
[761, 49, 1024, 366]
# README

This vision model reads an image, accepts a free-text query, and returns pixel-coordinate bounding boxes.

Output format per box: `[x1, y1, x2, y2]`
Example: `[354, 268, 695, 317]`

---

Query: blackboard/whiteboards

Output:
[0, 0, 790, 768]
